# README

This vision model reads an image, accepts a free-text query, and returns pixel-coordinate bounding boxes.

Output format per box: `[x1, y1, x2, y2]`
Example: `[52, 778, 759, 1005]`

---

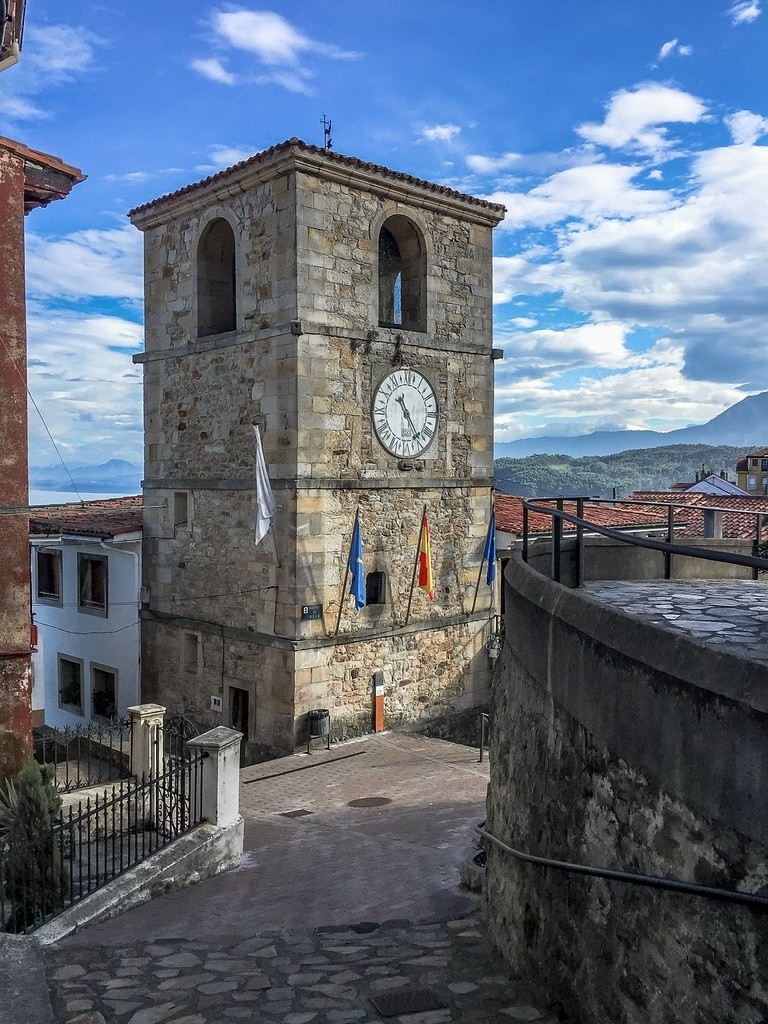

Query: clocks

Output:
[370, 367, 440, 459]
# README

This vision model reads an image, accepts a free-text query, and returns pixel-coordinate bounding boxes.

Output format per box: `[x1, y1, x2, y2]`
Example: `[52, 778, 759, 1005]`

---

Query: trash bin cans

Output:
[308, 709, 330, 736]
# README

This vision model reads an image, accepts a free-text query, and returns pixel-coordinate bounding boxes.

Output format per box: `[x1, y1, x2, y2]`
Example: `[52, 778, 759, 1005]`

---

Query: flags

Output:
[252, 425, 276, 544]
[349, 516, 366, 612]
[419, 515, 434, 600]
[484, 512, 496, 587]
